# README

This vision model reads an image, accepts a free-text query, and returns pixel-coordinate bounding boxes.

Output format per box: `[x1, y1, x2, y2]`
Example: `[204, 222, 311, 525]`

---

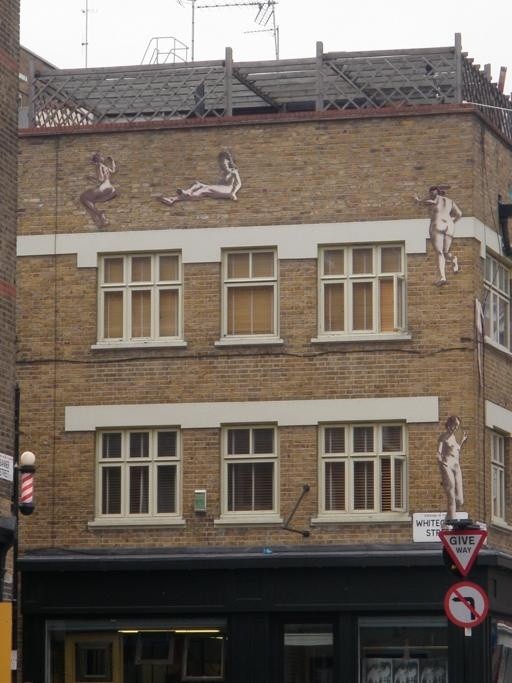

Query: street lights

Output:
[9, 383, 35, 681]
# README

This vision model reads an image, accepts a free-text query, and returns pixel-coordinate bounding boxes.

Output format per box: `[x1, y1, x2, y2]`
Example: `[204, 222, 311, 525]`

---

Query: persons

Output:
[158, 149, 242, 205]
[437, 416, 469, 527]
[80, 152, 117, 226]
[414, 186, 461, 286]
